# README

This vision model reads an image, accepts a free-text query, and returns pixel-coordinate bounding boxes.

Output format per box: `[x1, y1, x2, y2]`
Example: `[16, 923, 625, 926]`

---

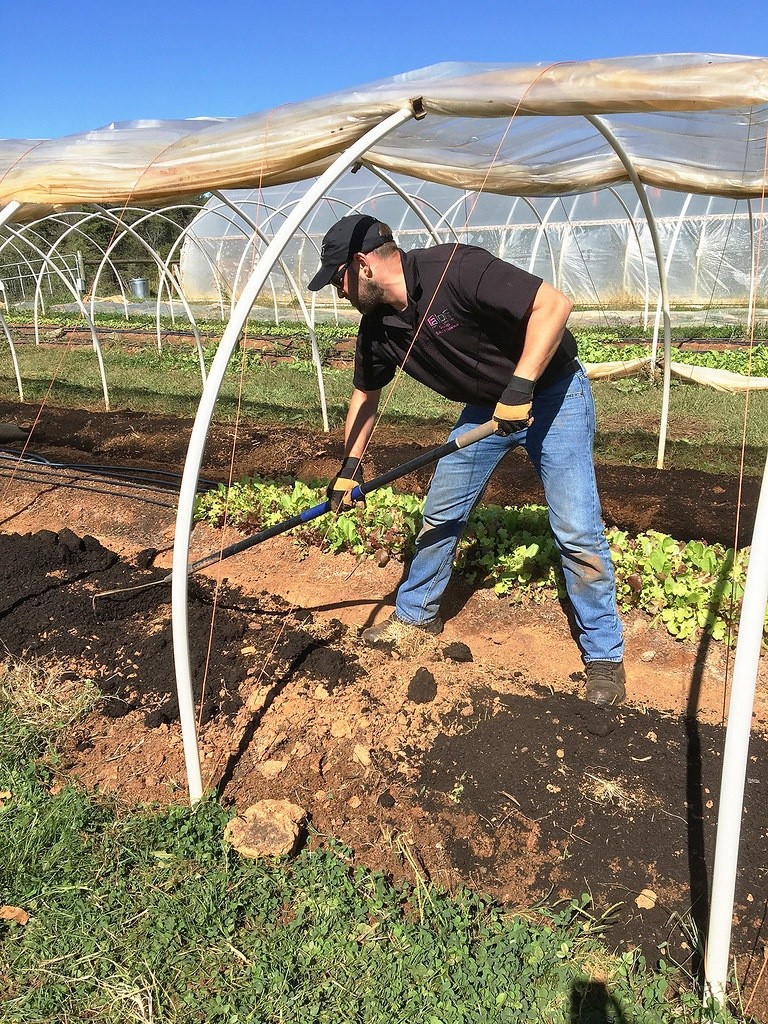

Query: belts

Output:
[535, 360, 580, 391]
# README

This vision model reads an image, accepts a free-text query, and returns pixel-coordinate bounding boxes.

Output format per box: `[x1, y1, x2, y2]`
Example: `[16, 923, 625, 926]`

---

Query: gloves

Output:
[492, 376, 536, 437]
[325, 458, 364, 511]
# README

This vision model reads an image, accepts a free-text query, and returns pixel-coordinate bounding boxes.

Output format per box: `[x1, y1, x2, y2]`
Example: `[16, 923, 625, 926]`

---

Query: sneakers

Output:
[585, 658, 626, 706]
[361, 612, 442, 640]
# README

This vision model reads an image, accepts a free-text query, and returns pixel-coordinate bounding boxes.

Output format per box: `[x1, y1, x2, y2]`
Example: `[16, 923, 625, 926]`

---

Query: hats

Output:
[307, 213, 394, 292]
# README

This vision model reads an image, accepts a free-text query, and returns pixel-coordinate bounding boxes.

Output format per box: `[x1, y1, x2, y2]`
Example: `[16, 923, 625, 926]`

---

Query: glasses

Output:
[330, 260, 352, 292]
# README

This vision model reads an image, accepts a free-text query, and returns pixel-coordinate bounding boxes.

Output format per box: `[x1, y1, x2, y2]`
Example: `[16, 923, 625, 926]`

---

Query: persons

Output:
[308, 213, 627, 707]
